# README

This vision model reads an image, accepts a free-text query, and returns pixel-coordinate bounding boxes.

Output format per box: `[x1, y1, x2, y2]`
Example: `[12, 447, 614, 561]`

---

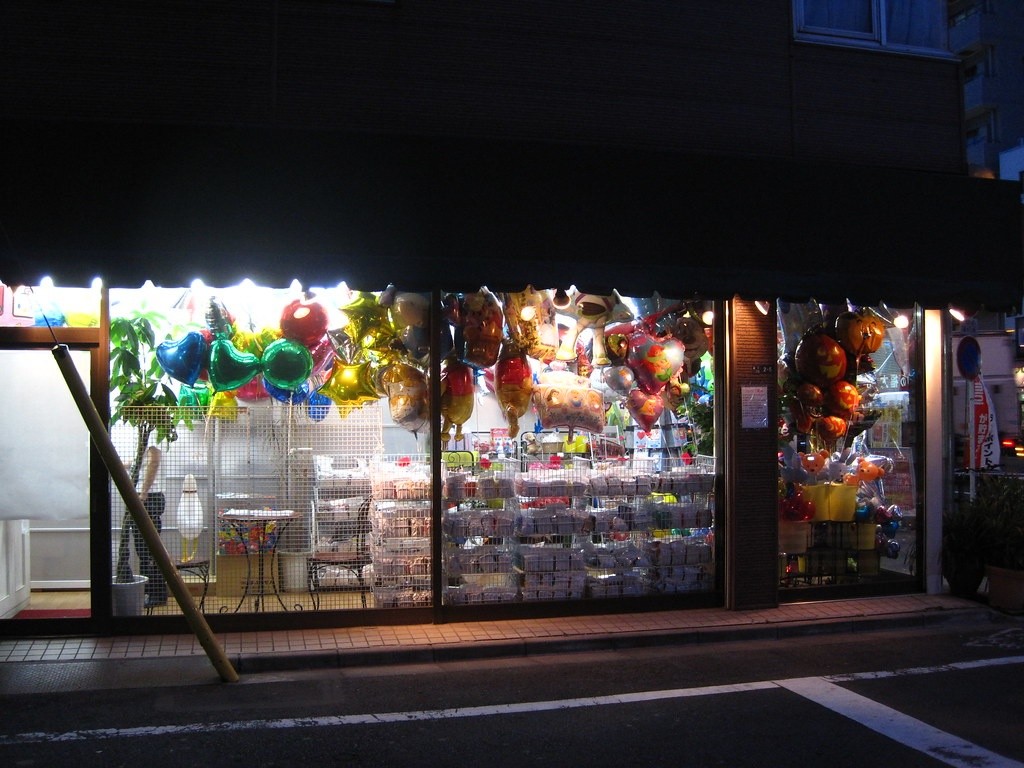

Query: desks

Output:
[219, 508, 303, 614]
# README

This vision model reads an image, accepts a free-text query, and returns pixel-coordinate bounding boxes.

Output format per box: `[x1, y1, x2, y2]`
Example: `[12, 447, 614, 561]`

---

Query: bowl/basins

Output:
[541, 441, 565, 453]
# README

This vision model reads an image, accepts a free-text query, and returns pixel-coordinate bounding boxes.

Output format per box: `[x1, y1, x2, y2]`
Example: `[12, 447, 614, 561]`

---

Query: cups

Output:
[576, 436, 587, 452]
[564, 435, 576, 452]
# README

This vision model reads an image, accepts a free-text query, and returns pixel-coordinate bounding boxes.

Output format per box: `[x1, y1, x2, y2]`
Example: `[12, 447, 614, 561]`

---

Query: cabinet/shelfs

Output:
[309, 451, 885, 610]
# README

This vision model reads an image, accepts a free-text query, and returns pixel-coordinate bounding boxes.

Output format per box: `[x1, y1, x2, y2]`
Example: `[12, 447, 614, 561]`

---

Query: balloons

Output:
[148, 283, 904, 560]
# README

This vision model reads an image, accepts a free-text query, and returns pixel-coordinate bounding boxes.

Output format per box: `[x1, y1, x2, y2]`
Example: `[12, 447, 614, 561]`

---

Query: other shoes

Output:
[145, 597, 167, 606]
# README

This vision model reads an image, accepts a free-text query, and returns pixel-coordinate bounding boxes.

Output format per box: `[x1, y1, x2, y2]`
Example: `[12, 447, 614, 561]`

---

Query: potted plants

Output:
[107, 311, 211, 616]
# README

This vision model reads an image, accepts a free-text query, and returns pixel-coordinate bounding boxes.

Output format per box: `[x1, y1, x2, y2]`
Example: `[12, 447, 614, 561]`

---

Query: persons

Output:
[131, 424, 168, 608]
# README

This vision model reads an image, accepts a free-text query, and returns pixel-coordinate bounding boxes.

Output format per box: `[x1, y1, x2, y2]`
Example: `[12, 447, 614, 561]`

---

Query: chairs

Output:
[147, 525, 210, 616]
[306, 494, 377, 610]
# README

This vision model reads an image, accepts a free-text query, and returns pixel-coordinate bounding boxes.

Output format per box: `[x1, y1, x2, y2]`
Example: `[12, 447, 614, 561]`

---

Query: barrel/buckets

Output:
[278, 548, 310, 592]
[829, 483, 857, 521]
[849, 523, 875, 550]
[800, 485, 829, 521]
[779, 520, 807, 554]
